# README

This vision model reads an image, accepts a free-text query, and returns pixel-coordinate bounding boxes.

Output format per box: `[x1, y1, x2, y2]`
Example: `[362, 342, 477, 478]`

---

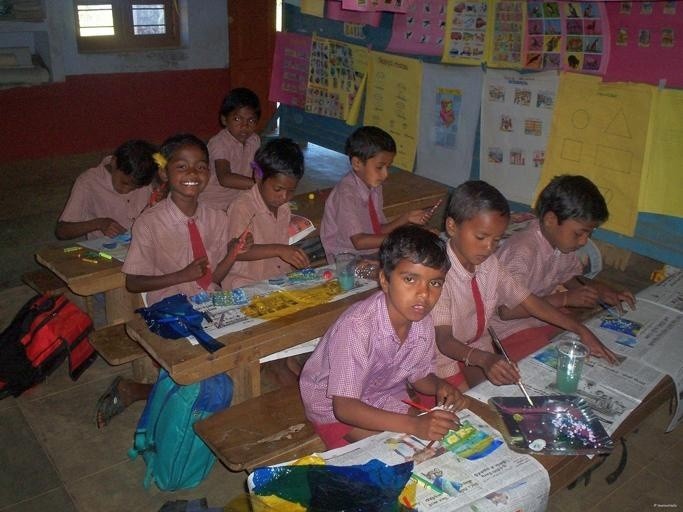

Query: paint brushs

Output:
[574, 276, 614, 314]
[487, 325, 533, 407]
[138, 183, 167, 215]
[232, 213, 256, 257]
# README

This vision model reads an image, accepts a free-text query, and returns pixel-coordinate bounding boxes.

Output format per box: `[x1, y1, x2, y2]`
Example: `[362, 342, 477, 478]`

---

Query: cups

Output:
[556, 340, 589, 393]
[334, 250, 358, 289]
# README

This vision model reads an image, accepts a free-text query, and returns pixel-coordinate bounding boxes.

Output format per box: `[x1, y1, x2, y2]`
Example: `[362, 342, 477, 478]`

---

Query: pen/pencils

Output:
[430, 199, 443, 214]
[411, 474, 448, 498]
[217, 314, 225, 328]
[401, 398, 463, 427]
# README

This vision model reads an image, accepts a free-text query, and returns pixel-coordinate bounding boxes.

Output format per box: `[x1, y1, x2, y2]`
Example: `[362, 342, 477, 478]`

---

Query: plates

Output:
[488, 394, 616, 455]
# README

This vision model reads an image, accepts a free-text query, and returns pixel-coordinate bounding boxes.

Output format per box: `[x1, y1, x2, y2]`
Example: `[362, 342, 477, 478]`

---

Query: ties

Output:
[368, 192, 382, 235]
[471, 276, 485, 337]
[188, 220, 213, 291]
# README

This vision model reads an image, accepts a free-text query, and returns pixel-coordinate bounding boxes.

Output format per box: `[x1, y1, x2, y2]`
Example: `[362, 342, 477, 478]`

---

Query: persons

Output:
[196, 86, 264, 213]
[211, 138, 312, 293]
[318, 125, 435, 263]
[488, 173, 638, 367]
[406, 179, 621, 413]
[55, 138, 164, 240]
[95, 132, 255, 429]
[298, 223, 470, 451]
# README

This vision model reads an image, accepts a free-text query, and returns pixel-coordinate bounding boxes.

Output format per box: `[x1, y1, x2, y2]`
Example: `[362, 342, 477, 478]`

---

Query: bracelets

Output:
[562, 288, 569, 309]
[463, 344, 474, 368]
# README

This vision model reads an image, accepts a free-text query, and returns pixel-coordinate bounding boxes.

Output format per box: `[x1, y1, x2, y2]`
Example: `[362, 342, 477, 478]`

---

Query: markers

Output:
[64, 246, 112, 264]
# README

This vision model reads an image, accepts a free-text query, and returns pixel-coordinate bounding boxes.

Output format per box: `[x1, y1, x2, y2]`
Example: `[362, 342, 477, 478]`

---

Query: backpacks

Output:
[127, 369, 233, 491]
[0, 292, 98, 400]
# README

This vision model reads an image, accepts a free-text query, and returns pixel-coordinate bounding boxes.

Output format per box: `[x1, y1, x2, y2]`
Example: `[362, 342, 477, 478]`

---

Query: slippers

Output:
[94, 376, 125, 430]
